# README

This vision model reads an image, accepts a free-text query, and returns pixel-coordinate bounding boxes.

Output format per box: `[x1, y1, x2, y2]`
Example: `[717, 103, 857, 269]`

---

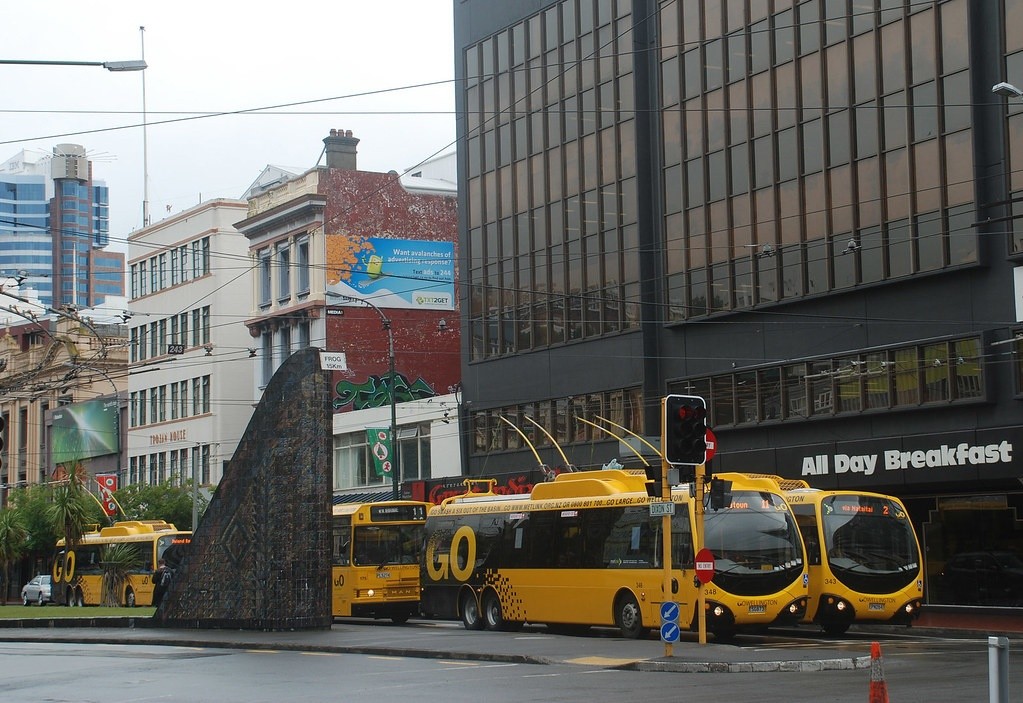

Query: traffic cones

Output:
[869, 642, 888, 703]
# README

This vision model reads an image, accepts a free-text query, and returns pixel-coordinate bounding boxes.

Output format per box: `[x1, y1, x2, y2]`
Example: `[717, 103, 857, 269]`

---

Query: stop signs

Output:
[706, 428, 716, 462]
[694, 548, 714, 584]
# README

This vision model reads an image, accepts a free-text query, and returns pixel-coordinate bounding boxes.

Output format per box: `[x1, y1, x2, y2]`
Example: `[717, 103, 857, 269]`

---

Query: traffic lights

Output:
[666, 395, 707, 466]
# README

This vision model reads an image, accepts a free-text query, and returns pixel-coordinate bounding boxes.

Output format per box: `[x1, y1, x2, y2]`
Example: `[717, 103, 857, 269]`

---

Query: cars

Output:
[20, 575, 51, 607]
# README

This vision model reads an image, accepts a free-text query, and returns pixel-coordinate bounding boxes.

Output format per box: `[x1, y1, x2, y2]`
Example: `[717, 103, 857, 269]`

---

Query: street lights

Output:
[324, 291, 399, 500]
[62, 360, 122, 520]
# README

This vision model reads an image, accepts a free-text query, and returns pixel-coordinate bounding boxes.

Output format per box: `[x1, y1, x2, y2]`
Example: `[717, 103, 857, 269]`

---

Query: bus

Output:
[332, 501, 434, 623]
[742, 472, 923, 635]
[50, 521, 193, 608]
[421, 470, 807, 640]
